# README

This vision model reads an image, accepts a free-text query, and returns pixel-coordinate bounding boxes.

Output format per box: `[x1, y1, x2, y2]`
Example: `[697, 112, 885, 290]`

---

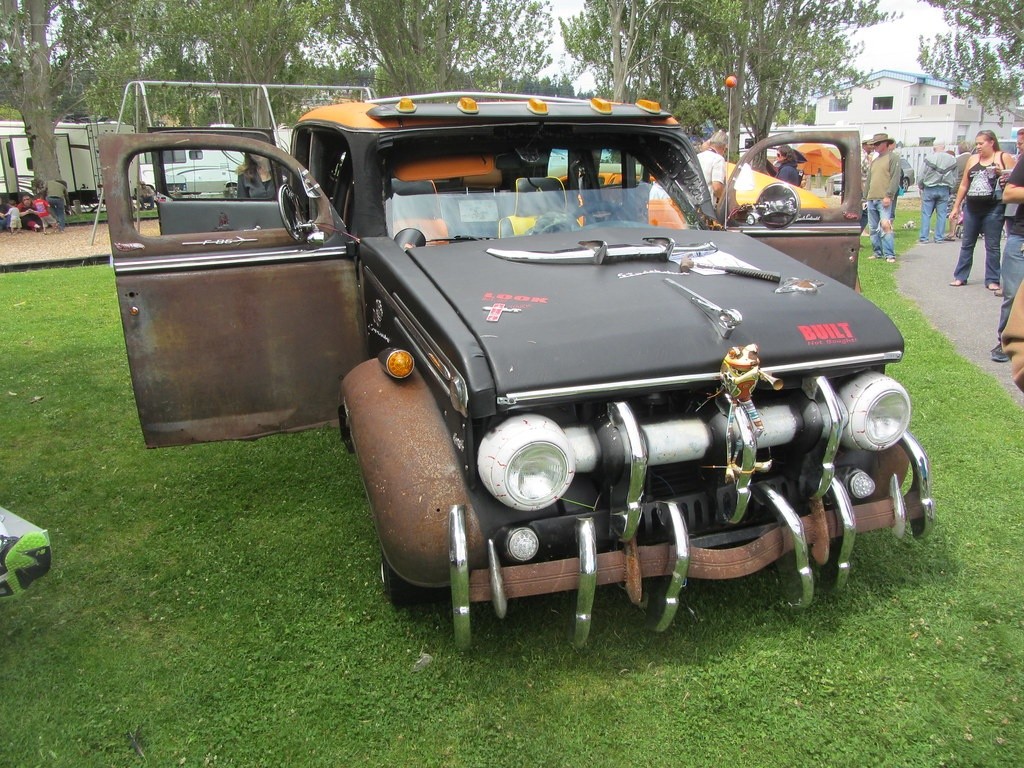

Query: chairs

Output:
[497, 176, 575, 239]
[389, 177, 450, 245]
[444, 169, 516, 239]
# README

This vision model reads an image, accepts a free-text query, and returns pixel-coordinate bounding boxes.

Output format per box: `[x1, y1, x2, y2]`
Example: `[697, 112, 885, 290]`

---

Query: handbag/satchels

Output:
[1000, 173, 1010, 189]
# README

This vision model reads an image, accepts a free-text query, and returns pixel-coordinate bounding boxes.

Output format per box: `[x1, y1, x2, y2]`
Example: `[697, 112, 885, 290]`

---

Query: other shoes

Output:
[934, 240, 944, 244]
[921, 240, 929, 243]
[988, 282, 1000, 290]
[950, 279, 966, 286]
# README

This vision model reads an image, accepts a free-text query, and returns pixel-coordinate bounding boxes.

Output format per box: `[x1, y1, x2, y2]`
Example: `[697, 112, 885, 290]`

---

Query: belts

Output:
[50, 197, 59, 198]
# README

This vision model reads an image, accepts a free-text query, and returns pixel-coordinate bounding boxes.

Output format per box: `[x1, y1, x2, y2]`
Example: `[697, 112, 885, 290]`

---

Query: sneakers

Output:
[0, 531, 51, 597]
[991, 344, 1009, 362]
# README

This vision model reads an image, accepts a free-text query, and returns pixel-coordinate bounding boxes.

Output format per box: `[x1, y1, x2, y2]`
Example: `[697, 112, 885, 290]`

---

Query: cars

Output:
[99, 79, 934, 609]
[824, 159, 914, 195]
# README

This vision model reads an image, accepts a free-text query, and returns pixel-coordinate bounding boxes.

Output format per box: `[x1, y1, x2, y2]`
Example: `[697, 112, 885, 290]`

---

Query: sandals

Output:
[944, 234, 955, 241]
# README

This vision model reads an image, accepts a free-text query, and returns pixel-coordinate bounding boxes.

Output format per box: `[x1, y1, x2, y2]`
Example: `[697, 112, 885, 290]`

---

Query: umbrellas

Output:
[793, 143, 842, 176]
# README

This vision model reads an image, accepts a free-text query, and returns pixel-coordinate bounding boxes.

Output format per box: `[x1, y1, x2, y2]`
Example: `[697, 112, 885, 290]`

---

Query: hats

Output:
[861, 135, 873, 144]
[867, 134, 894, 144]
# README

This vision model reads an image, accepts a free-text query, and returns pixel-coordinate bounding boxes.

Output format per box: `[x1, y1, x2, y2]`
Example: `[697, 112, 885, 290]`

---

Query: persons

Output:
[0, 180, 71, 232]
[136, 178, 154, 211]
[696, 129, 1024, 393]
[236, 150, 279, 198]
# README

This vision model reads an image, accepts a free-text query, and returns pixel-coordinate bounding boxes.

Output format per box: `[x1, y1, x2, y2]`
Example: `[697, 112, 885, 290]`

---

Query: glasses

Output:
[873, 142, 881, 146]
[777, 153, 781, 157]
